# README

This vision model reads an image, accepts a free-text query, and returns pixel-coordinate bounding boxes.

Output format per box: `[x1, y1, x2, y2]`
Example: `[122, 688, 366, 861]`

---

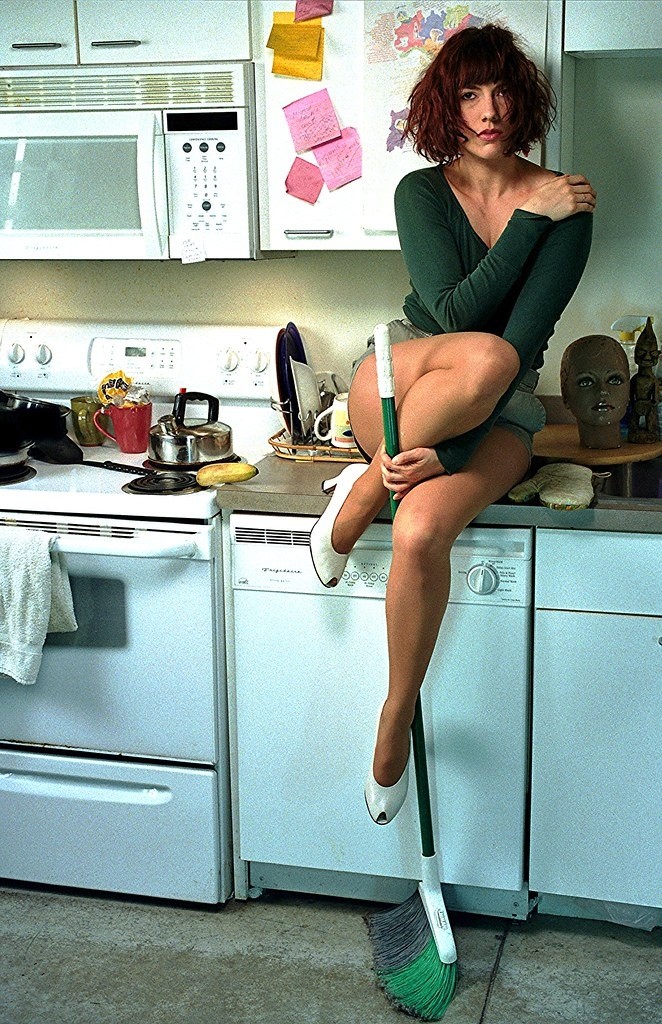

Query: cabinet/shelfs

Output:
[528, 528, 661, 932]
[249, 0, 575, 251]
[0, 0, 252, 67]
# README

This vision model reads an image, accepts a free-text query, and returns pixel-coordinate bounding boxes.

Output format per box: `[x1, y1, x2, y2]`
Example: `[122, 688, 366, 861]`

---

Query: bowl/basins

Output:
[290, 355, 322, 434]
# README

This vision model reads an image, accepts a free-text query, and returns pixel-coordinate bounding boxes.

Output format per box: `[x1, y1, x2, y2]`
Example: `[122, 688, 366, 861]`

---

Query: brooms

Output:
[356, 319, 463, 1022]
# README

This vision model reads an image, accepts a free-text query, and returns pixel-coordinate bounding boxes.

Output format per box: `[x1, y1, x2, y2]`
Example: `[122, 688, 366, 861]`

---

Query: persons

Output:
[560, 335, 630, 449]
[307, 22, 599, 824]
[627, 317, 660, 445]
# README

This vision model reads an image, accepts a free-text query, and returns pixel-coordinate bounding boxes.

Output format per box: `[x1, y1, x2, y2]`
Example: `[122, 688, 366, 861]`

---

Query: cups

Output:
[314, 393, 362, 450]
[93, 402, 152, 454]
[71, 396, 110, 446]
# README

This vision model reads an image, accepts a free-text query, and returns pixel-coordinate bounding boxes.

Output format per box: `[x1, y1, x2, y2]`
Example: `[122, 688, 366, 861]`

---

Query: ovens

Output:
[0, 509, 225, 904]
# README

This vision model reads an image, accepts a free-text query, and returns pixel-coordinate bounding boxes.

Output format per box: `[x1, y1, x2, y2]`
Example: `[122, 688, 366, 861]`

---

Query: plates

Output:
[269, 321, 306, 439]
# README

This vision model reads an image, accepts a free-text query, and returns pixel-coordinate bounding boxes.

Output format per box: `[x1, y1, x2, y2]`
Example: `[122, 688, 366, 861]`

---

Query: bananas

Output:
[196, 461, 260, 486]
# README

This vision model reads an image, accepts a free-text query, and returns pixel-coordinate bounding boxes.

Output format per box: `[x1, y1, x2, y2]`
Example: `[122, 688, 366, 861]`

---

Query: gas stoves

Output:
[0, 422, 242, 499]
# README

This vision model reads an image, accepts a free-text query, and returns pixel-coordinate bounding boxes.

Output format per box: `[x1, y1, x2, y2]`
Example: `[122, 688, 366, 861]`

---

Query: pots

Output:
[0, 388, 62, 416]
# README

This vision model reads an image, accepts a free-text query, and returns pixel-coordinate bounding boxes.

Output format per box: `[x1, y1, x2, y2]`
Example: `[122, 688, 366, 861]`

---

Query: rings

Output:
[582, 194, 586, 203]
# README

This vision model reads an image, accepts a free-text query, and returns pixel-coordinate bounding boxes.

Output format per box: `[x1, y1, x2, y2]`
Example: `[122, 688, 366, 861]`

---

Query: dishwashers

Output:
[228, 511, 534, 892]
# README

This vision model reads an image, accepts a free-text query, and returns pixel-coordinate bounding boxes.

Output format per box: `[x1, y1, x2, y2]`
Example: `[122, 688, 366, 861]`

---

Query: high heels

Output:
[365, 698, 410, 825]
[310, 464, 373, 587]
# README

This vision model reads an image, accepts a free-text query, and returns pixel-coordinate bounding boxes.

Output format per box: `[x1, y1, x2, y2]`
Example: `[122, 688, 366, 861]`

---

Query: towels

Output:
[0, 526, 81, 686]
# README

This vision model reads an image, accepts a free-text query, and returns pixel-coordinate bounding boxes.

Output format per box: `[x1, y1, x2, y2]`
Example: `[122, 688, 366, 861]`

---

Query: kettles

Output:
[148, 392, 233, 463]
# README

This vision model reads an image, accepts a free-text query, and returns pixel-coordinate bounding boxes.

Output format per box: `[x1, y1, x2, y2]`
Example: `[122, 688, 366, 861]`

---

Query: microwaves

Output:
[0, 109, 258, 262]
